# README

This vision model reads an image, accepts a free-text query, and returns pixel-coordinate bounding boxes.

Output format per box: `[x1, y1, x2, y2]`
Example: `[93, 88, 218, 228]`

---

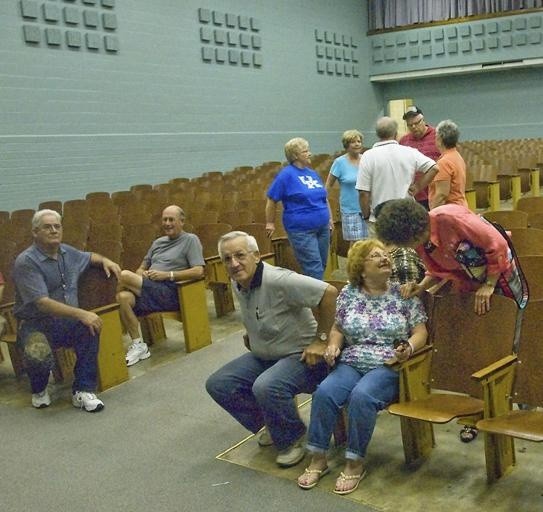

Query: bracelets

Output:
[358, 211, 372, 221]
[329, 215, 333, 218]
[484, 280, 496, 288]
[170, 270, 174, 283]
[405, 340, 414, 356]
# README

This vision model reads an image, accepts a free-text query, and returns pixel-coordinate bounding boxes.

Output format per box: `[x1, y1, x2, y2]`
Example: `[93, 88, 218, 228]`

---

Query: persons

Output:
[324, 128, 372, 257]
[396, 104, 441, 214]
[12, 209, 123, 412]
[263, 136, 335, 281]
[371, 200, 427, 286]
[295, 239, 429, 496]
[115, 205, 205, 367]
[354, 115, 440, 235]
[375, 199, 529, 444]
[428, 120, 469, 216]
[204, 231, 340, 468]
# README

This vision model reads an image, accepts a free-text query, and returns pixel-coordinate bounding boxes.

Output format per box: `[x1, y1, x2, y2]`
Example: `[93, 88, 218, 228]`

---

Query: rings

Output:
[481, 300, 486, 304]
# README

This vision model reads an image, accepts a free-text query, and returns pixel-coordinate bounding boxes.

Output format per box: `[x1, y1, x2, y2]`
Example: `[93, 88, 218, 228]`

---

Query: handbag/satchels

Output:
[455, 214, 530, 310]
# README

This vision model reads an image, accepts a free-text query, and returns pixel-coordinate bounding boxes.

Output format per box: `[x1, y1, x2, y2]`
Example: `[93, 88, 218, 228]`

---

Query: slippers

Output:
[297, 465, 329, 488]
[333, 467, 368, 496]
[461, 424, 478, 444]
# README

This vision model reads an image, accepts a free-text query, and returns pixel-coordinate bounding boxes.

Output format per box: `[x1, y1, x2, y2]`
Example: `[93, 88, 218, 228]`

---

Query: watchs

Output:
[314, 330, 329, 342]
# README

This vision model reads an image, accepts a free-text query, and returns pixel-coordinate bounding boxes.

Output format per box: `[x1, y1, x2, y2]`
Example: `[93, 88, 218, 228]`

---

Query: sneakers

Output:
[122, 342, 152, 366]
[69, 388, 105, 412]
[275, 438, 305, 467]
[256, 425, 273, 447]
[32, 387, 50, 410]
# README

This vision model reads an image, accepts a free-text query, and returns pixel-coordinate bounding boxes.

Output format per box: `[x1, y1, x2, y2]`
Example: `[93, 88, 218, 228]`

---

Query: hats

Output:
[403, 106, 423, 119]
